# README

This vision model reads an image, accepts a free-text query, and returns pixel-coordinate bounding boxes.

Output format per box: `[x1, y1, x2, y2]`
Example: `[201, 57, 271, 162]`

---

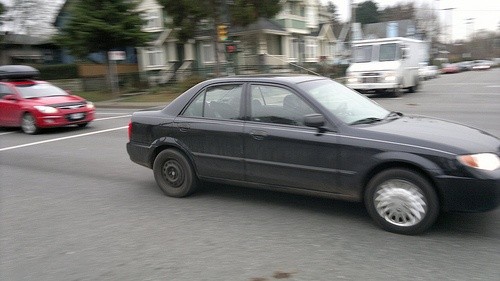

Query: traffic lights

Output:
[218, 24, 229, 43]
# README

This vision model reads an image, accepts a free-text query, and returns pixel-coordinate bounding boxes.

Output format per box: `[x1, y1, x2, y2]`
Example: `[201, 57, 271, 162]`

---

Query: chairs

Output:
[193, 101, 216, 119]
[281, 94, 312, 126]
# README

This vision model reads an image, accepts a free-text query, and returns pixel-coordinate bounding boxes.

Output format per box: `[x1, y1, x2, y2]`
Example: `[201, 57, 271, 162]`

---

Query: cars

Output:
[420, 55, 500, 79]
[1, 66, 96, 134]
[125, 75, 500, 236]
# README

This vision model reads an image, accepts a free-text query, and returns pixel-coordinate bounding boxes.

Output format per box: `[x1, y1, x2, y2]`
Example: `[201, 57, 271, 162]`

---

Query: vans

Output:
[343, 38, 431, 93]
